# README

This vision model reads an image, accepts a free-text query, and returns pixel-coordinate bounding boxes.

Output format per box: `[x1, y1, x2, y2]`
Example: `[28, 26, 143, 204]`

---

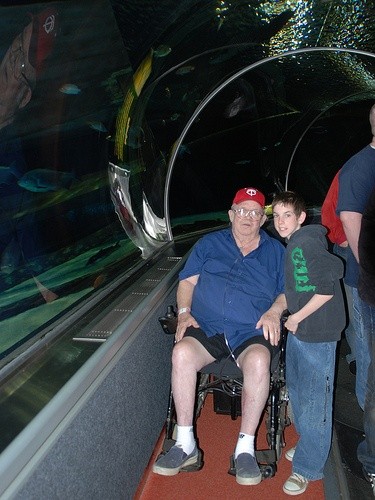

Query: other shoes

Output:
[233, 447, 262, 485]
[362, 467, 375, 496]
[152, 438, 199, 476]
[348, 360, 357, 377]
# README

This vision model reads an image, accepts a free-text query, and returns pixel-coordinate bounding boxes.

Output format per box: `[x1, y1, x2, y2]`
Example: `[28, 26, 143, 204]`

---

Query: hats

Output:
[28, 4, 59, 80]
[233, 187, 266, 209]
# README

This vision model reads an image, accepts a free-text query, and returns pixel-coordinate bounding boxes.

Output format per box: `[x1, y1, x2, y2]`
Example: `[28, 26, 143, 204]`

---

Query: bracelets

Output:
[178, 308, 191, 315]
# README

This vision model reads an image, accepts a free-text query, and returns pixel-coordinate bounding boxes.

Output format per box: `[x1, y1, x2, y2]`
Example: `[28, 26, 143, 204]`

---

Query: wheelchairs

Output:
[155, 306, 289, 480]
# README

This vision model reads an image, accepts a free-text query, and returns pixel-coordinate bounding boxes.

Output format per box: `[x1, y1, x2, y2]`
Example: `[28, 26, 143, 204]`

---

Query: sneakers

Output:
[285, 446, 296, 461]
[283, 472, 308, 496]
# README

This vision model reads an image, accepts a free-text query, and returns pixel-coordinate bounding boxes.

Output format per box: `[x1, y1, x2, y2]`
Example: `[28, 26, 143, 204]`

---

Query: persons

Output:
[322, 105, 375, 497]
[153, 188, 288, 485]
[272, 191, 346, 495]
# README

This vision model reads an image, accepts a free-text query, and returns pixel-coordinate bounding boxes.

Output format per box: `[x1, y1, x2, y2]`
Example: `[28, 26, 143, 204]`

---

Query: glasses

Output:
[230, 208, 265, 221]
[10, 25, 35, 91]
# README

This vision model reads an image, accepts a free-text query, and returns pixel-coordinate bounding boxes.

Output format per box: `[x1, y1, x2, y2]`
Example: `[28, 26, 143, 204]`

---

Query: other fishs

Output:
[177, 66, 195, 73]
[227, 96, 243, 117]
[151, 44, 172, 57]
[59, 83, 81, 95]
[87, 121, 106, 132]
[17, 167, 73, 194]
